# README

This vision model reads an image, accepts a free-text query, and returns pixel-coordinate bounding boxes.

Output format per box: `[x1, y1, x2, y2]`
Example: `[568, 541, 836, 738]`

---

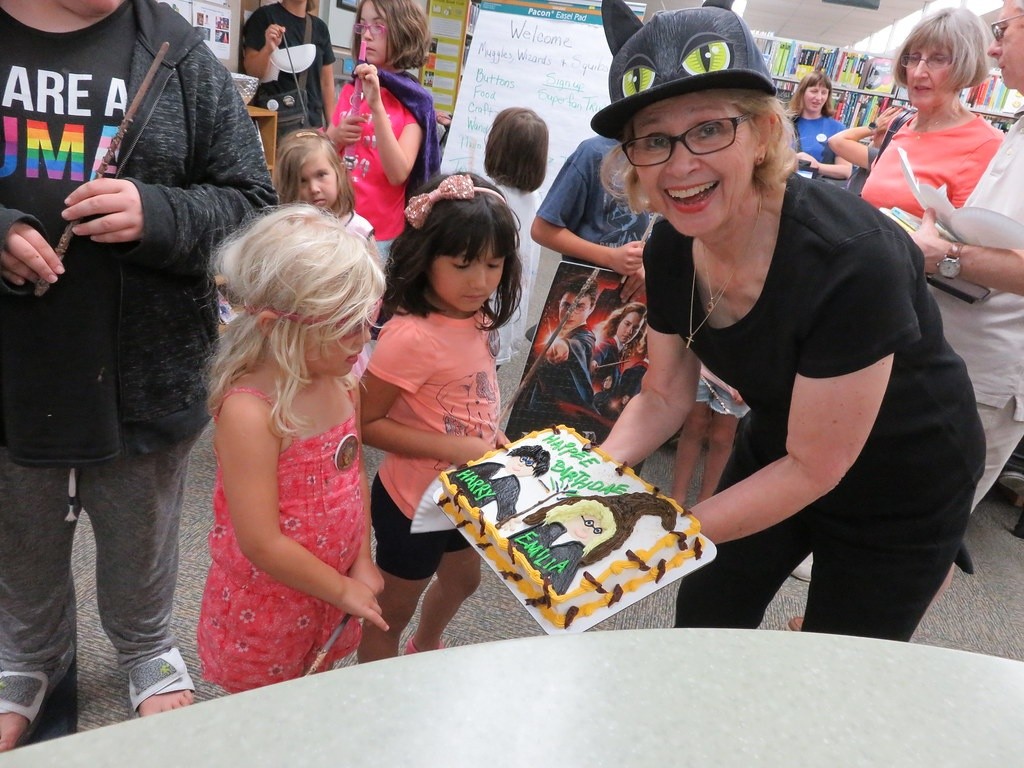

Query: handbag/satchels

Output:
[258, 87, 310, 136]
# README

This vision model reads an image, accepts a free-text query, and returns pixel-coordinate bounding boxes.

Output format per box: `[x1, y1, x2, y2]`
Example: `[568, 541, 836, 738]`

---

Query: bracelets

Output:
[869, 123, 876, 130]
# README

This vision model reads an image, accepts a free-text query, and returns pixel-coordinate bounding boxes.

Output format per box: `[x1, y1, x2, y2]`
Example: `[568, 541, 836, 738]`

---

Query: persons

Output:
[531, 135, 661, 303]
[357, 171, 522, 664]
[598, 0, 986, 642]
[785, 72, 853, 178]
[243, 0, 335, 147]
[526, 274, 650, 427]
[483, 107, 548, 372]
[791, 5, 1002, 581]
[196, 203, 389, 695]
[273, 130, 386, 384]
[325, 0, 440, 234]
[788, 0, 1024, 633]
[0, 0, 278, 751]
[672, 361, 751, 508]
[828, 105, 906, 198]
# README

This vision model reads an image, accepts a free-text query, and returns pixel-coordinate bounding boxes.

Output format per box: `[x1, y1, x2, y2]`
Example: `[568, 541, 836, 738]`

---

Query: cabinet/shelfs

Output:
[770, 74, 1016, 145]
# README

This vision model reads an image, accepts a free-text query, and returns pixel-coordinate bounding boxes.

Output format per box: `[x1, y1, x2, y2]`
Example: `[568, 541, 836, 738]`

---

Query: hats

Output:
[589, 0, 777, 139]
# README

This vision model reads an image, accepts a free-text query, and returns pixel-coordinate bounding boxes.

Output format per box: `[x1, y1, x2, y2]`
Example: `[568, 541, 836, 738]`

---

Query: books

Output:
[754, 38, 1024, 132]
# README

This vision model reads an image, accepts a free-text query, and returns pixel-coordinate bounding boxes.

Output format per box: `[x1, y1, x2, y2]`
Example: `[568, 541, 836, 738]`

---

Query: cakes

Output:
[437, 423, 704, 630]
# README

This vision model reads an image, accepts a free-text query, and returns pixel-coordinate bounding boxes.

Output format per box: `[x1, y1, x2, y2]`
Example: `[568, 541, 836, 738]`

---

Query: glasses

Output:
[991, 14, 1024, 42]
[620, 112, 757, 166]
[353, 23, 386, 36]
[901, 53, 952, 69]
[249, 297, 384, 340]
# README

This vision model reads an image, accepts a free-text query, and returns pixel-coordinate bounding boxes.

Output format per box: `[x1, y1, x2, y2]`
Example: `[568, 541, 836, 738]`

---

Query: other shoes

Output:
[789, 616, 804, 630]
[791, 551, 814, 582]
[404, 636, 445, 657]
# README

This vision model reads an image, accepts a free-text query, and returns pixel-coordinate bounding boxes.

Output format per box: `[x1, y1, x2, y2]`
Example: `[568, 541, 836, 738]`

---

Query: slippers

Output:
[0, 641, 74, 752]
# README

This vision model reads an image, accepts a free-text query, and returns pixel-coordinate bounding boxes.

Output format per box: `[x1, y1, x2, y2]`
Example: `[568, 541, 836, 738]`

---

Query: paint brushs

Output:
[618, 212, 658, 293]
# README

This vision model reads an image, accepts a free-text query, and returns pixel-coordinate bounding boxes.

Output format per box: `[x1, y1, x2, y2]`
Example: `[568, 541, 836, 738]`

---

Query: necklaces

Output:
[686, 194, 762, 348]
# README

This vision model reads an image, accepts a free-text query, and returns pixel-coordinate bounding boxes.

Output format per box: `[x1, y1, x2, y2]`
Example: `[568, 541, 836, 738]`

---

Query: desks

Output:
[247, 104, 279, 183]
[0, 627, 1024, 768]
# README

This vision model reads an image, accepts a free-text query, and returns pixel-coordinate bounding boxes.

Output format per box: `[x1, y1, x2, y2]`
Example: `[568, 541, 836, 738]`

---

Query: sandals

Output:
[128, 646, 196, 718]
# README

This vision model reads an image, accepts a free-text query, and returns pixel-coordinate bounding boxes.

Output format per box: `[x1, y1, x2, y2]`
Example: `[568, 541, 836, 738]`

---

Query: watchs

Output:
[937, 242, 963, 279]
